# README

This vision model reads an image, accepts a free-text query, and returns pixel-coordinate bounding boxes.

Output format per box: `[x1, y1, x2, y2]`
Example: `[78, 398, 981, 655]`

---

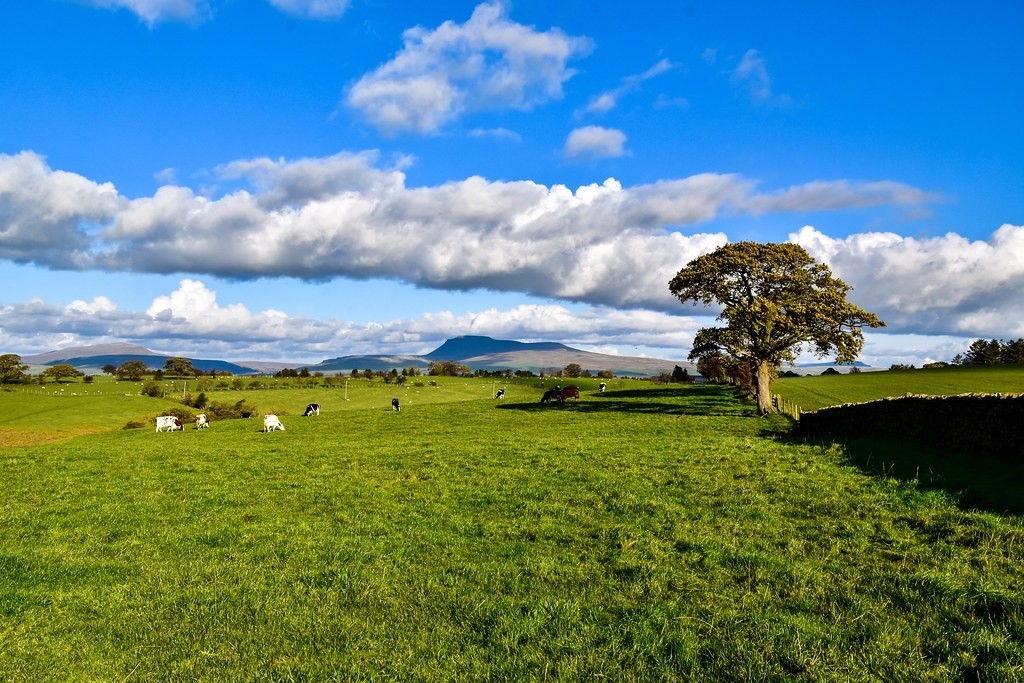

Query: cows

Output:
[263, 415, 286, 432]
[304, 403, 321, 417]
[155, 415, 184, 432]
[392, 398, 401, 412]
[194, 413, 210, 430]
[495, 388, 505, 399]
[539, 388, 579, 404]
[599, 382, 606, 393]
